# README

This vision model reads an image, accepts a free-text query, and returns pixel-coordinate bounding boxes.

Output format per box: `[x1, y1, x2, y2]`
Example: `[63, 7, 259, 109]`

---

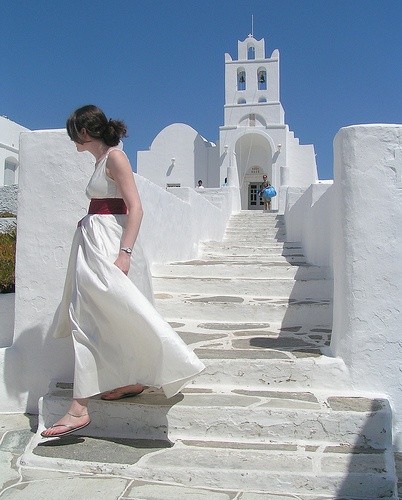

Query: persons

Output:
[260, 175, 271, 210]
[39, 104, 152, 438]
[195, 180, 204, 188]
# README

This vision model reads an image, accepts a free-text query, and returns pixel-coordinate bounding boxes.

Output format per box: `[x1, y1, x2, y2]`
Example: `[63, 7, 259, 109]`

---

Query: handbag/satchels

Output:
[264, 186, 276, 197]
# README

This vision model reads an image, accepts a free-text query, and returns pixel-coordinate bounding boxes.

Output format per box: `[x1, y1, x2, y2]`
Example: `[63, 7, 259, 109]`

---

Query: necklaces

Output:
[94, 154, 104, 165]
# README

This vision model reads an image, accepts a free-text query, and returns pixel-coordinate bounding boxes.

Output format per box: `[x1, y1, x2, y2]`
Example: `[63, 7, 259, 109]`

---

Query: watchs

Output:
[120, 247, 133, 254]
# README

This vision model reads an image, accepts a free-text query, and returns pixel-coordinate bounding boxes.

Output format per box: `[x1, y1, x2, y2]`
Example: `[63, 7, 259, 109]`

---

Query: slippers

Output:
[101, 386, 144, 400]
[41, 419, 91, 436]
